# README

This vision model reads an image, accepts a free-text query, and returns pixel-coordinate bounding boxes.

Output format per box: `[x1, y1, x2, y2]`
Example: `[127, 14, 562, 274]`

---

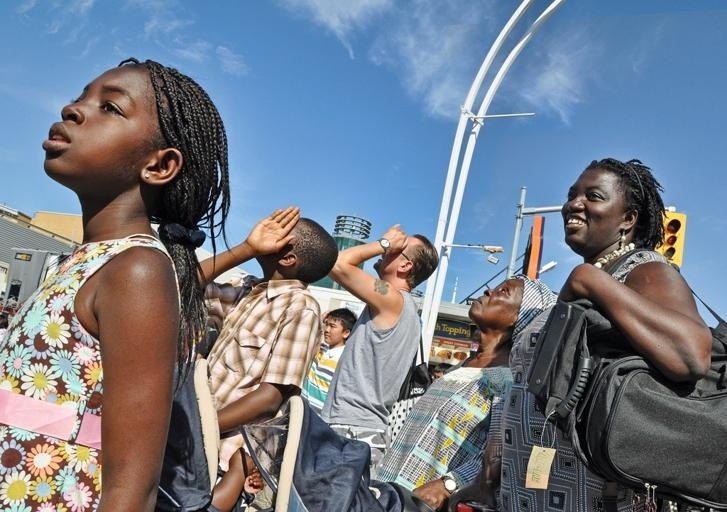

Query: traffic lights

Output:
[652, 208, 689, 266]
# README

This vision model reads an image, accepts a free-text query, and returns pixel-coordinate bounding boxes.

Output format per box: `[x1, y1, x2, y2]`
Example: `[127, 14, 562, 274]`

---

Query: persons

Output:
[0, 63, 231, 512]
[194, 207, 323, 472]
[200, 449, 265, 512]
[0, 291, 23, 328]
[370, 276, 559, 512]
[434, 362, 452, 380]
[300, 310, 357, 415]
[387, 394, 414, 446]
[328, 222, 439, 465]
[501, 157, 711, 511]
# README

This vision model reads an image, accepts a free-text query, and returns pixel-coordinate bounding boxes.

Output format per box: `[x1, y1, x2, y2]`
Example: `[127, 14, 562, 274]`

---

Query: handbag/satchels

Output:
[387, 361, 432, 442]
[527, 297, 726, 512]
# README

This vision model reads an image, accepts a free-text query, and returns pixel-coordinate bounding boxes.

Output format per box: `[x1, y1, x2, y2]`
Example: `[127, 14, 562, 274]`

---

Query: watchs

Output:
[377, 235, 391, 254]
[440, 472, 461, 498]
